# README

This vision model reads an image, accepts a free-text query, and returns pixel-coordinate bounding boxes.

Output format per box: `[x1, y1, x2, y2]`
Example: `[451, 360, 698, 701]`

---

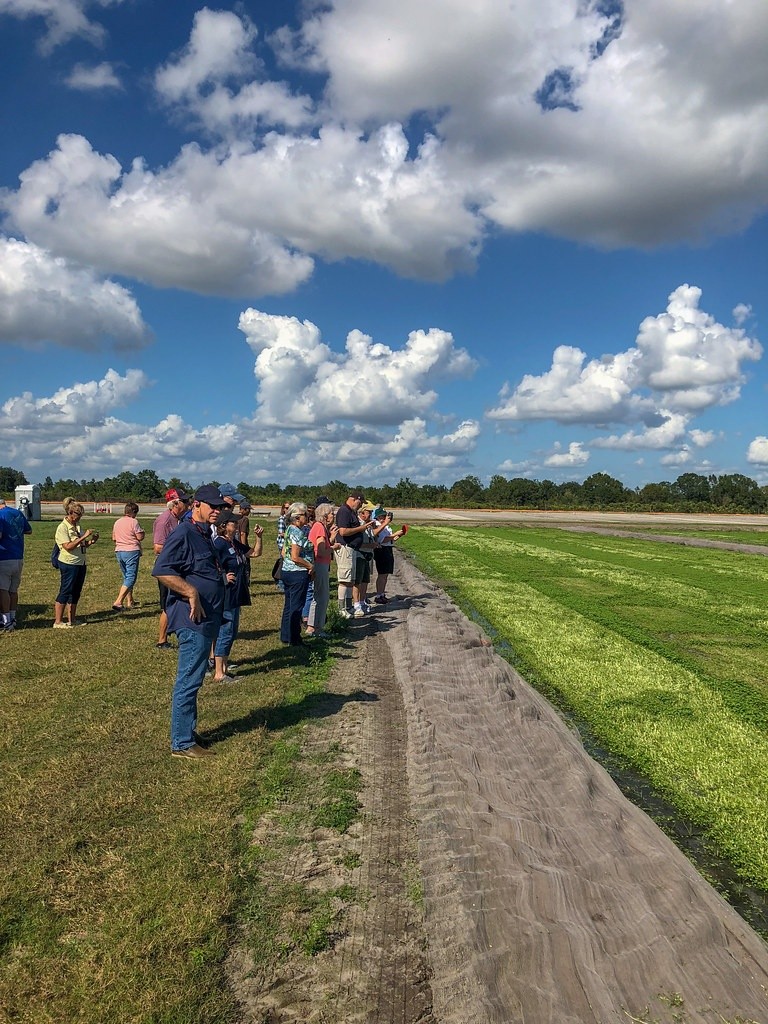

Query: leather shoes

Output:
[194, 731, 217, 745]
[172, 744, 217, 761]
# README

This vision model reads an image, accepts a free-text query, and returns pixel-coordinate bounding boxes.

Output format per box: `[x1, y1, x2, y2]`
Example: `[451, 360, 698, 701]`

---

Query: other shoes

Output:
[154, 641, 179, 651]
[283, 592, 389, 649]
[204, 656, 245, 685]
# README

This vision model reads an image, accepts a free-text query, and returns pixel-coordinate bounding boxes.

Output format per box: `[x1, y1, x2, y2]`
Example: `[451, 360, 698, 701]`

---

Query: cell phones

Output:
[387, 512, 393, 521]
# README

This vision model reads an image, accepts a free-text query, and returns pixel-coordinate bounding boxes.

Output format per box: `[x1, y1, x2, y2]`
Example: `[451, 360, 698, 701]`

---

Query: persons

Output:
[52, 496, 99, 629]
[151, 485, 233, 759]
[280, 503, 312, 648]
[209, 481, 255, 549]
[276, 496, 339, 627]
[111, 501, 145, 613]
[333, 492, 404, 618]
[153, 487, 193, 649]
[307, 503, 342, 637]
[212, 510, 264, 684]
[0, 499, 33, 631]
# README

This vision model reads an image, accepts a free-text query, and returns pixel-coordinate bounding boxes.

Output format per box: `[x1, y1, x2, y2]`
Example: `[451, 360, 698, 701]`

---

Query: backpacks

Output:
[51, 543, 61, 569]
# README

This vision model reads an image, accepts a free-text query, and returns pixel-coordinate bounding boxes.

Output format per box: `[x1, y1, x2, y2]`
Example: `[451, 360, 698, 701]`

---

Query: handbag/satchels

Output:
[359, 550, 373, 560]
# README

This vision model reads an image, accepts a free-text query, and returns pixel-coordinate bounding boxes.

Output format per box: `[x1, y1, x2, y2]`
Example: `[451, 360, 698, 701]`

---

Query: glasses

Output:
[225, 496, 238, 505]
[74, 511, 83, 516]
[201, 500, 224, 511]
[284, 507, 289, 510]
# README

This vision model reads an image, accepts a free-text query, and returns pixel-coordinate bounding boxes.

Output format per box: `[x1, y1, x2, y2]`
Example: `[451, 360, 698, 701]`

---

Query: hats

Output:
[374, 507, 387, 519]
[195, 484, 232, 509]
[315, 496, 334, 508]
[165, 488, 194, 503]
[213, 511, 244, 526]
[217, 482, 246, 501]
[358, 500, 377, 512]
[240, 502, 255, 510]
[351, 492, 368, 504]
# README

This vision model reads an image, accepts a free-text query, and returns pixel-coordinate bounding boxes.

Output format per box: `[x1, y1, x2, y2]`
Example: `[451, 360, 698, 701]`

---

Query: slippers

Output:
[112, 605, 130, 613]
[127, 601, 141, 609]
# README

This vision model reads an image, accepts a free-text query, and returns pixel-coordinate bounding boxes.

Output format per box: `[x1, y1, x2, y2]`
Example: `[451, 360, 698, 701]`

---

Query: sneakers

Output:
[51, 619, 87, 629]
[0, 617, 17, 631]
